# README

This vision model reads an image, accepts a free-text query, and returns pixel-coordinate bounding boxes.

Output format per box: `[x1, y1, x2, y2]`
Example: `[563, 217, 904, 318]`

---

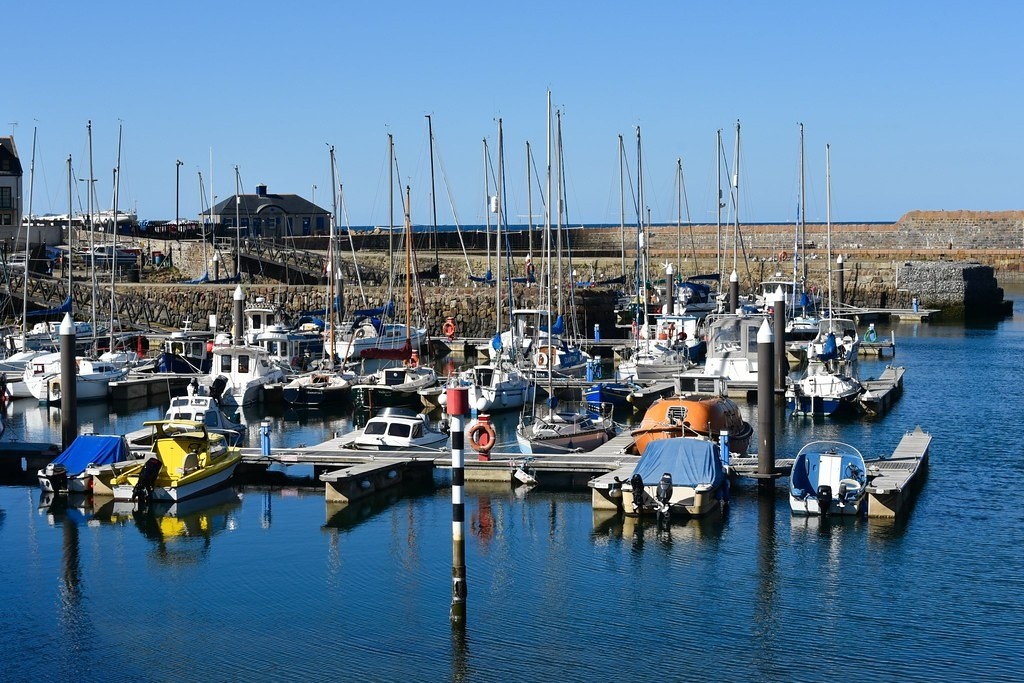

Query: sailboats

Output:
[3, 88, 861, 454]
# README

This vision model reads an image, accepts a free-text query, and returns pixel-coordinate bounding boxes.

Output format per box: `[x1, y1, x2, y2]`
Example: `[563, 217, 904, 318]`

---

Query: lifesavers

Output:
[533, 352, 548, 366]
[355, 329, 365, 338]
[403, 356, 419, 368]
[326, 330, 335, 340]
[779, 252, 787, 261]
[632, 322, 639, 336]
[443, 322, 455, 336]
[468, 422, 496, 452]
[526, 262, 534, 275]
[654, 308, 661, 314]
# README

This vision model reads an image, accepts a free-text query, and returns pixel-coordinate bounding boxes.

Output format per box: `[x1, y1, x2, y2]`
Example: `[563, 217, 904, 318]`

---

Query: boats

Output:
[352, 406, 447, 452]
[161, 395, 246, 434]
[631, 387, 754, 458]
[788, 439, 867, 519]
[107, 418, 245, 504]
[586, 436, 726, 522]
[38, 433, 136, 495]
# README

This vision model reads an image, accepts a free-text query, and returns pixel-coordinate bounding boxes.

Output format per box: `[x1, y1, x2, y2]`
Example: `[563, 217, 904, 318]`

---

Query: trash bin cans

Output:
[127, 270, 140, 282]
